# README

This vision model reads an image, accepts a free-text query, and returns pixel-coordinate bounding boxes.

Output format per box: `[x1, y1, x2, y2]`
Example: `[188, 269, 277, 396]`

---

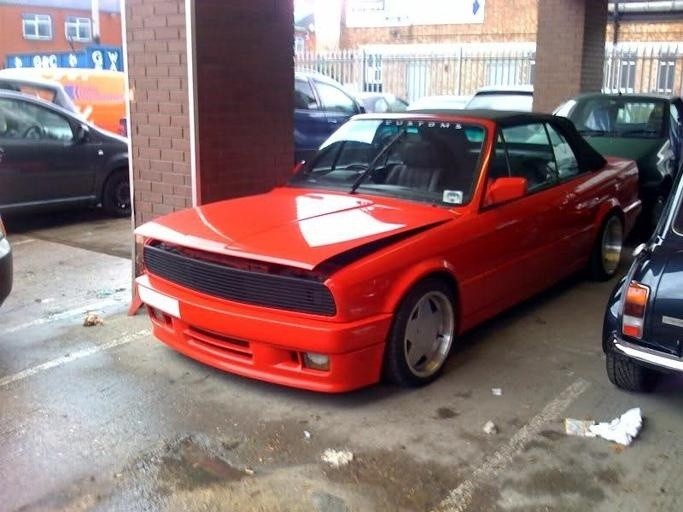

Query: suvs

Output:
[293, 68, 366, 169]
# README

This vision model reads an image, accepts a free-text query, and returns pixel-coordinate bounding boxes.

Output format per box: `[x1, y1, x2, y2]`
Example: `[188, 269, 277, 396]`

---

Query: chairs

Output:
[385, 139, 441, 192]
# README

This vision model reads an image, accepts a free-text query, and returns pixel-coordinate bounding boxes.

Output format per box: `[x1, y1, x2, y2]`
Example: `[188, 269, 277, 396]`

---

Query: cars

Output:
[600, 162, 683, 393]
[463, 86, 536, 114]
[405, 94, 472, 111]
[0, 66, 131, 307]
[523, 90, 683, 240]
[358, 93, 409, 114]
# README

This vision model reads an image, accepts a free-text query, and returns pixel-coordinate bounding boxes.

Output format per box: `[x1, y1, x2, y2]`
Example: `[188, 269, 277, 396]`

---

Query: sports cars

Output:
[135, 108, 643, 394]
